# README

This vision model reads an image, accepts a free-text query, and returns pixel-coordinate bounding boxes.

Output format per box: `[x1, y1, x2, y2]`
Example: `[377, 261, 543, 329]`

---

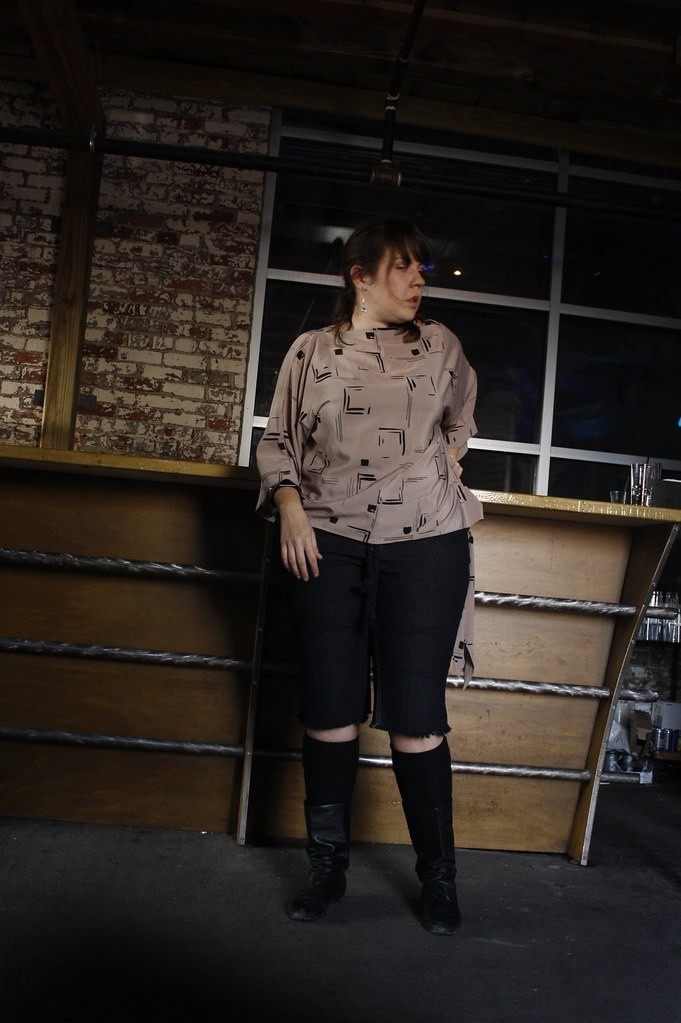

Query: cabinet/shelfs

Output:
[625, 640, 681, 760]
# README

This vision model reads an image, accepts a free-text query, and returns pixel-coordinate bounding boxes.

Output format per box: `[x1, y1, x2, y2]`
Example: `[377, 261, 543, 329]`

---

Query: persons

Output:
[253, 217, 484, 933]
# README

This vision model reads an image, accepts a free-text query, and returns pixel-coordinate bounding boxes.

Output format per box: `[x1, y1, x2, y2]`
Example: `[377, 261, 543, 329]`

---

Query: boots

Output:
[286, 800, 351, 919]
[390, 798, 461, 935]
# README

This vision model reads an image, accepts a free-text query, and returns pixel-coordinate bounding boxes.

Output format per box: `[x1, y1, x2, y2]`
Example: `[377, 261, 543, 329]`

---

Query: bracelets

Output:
[268, 479, 304, 508]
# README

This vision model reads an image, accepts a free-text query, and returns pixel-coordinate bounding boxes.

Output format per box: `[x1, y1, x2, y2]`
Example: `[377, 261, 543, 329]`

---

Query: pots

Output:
[605, 751, 633, 772]
[652, 727, 679, 752]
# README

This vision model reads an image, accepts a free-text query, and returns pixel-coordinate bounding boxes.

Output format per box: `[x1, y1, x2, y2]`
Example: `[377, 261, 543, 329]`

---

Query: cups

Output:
[635, 591, 681, 642]
[610, 491, 626, 504]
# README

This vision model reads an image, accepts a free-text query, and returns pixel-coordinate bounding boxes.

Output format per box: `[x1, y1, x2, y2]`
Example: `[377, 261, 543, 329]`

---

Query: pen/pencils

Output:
[629, 455, 662, 506]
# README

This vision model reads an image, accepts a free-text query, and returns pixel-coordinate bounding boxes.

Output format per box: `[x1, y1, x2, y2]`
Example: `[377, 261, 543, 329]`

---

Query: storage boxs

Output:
[651, 700, 681, 731]
[629, 710, 654, 757]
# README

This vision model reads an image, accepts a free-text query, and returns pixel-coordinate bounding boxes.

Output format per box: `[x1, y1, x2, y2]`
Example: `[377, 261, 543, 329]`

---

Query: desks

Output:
[0, 443, 681, 867]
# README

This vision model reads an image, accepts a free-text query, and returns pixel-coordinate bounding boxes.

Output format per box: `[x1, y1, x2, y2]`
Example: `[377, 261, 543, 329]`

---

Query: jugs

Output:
[630, 462, 662, 507]
